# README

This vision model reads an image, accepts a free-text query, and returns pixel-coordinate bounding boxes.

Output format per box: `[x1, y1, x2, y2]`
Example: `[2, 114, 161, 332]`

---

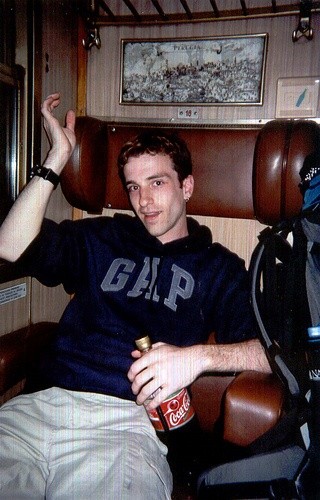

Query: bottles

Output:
[307, 325, 320, 419]
[135, 335, 223, 486]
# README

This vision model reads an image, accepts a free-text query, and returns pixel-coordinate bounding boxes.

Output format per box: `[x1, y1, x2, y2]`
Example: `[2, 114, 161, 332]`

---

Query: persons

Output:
[0, 93, 273, 500]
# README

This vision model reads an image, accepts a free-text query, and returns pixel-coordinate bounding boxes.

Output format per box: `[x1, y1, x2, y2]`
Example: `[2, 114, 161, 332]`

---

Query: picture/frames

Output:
[119, 33, 269, 108]
[276, 76, 318, 118]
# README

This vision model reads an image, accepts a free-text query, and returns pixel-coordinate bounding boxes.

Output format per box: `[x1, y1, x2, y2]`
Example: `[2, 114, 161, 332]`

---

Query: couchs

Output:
[0, 112, 319, 456]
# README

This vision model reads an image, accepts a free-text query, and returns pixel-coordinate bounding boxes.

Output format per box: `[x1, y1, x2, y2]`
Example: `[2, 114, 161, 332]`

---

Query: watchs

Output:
[29, 163, 61, 193]
